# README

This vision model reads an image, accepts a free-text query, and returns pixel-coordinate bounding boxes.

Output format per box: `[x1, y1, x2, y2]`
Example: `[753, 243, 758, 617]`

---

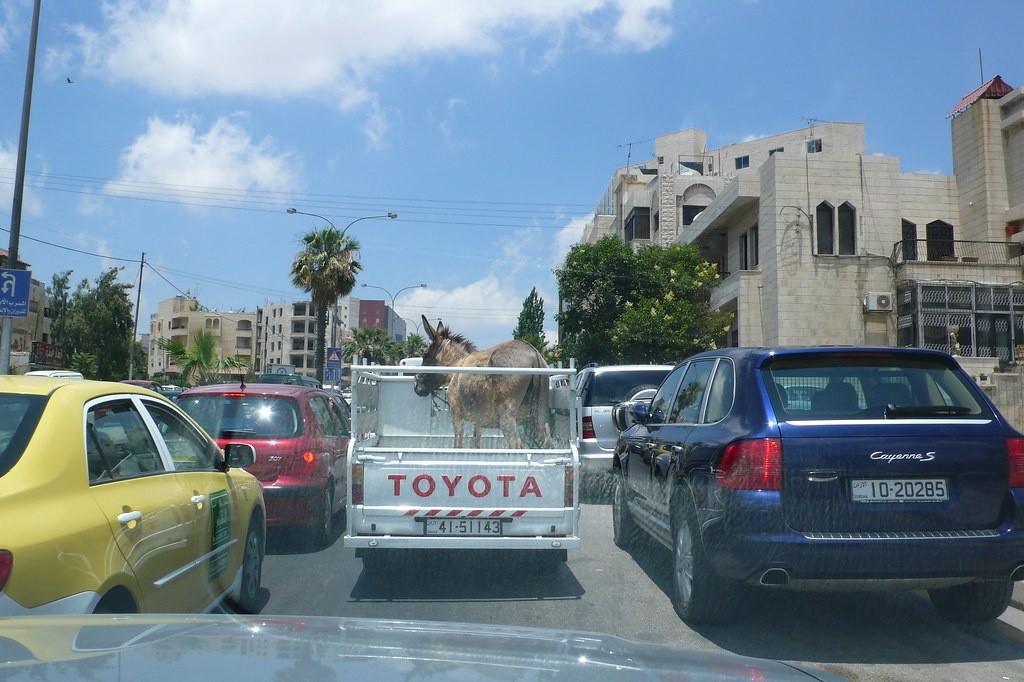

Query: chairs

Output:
[823, 382, 860, 411]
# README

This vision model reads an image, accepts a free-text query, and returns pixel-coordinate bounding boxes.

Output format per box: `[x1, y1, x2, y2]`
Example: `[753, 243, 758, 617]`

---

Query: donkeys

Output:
[414, 315, 559, 448]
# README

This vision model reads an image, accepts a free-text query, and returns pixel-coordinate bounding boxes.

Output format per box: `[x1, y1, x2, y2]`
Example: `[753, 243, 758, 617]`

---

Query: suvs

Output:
[575, 360, 682, 472]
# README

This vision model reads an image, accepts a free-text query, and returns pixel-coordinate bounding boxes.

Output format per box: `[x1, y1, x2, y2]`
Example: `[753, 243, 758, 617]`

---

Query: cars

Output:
[607, 343, 1023, 626]
[171, 383, 352, 545]
[118, 380, 185, 405]
[322, 385, 352, 408]
[0, 375, 269, 638]
[783, 385, 867, 411]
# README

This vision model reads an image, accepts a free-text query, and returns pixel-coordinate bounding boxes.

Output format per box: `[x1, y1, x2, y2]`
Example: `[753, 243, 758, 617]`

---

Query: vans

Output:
[260, 373, 323, 389]
[24, 370, 84, 380]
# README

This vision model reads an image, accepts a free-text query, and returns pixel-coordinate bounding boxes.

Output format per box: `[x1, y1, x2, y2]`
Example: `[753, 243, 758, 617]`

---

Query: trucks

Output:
[347, 357, 581, 562]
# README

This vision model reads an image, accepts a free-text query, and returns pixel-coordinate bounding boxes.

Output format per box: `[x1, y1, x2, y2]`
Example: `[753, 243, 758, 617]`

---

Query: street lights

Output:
[395, 317, 443, 336]
[286, 206, 398, 377]
[359, 283, 427, 339]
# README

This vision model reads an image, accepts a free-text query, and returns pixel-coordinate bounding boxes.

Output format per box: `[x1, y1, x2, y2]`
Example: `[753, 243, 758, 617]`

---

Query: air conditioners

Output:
[863, 291, 893, 313]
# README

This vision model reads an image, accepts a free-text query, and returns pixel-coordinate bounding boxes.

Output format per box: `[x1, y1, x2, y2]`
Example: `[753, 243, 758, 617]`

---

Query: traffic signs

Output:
[0, 269, 33, 319]
